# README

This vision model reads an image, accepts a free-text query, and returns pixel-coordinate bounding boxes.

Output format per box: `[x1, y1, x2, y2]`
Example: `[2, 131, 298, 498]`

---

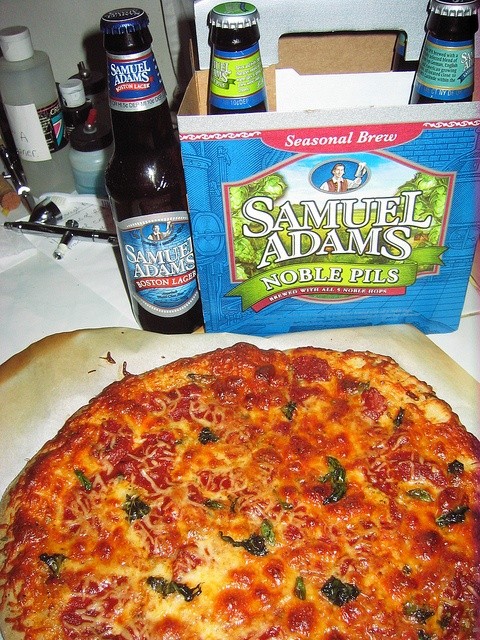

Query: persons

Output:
[320, 163, 363, 192]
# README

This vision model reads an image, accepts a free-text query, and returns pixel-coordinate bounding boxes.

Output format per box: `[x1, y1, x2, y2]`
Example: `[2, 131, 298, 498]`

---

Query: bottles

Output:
[58, 78, 92, 137]
[408, 0, 480, 103]
[99, 6, 203, 334]
[69, 109, 114, 198]
[0, 25, 75, 198]
[206, 2, 268, 113]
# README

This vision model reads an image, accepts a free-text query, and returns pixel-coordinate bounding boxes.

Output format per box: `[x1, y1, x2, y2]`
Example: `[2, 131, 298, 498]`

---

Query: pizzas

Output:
[0, 341, 479, 640]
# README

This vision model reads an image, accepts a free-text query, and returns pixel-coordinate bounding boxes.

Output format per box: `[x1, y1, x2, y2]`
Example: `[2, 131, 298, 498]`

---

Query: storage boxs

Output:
[176, 59, 480, 334]
